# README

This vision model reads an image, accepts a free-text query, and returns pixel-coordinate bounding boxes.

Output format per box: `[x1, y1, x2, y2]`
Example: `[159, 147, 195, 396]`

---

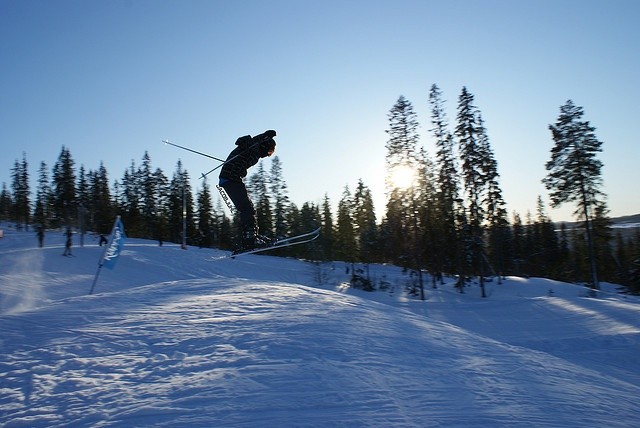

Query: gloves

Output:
[265, 129, 276, 138]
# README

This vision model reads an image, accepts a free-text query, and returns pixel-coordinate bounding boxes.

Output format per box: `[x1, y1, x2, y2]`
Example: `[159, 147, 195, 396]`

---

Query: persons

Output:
[63, 229, 78, 256]
[158, 239, 166, 248]
[33, 227, 48, 249]
[217, 126, 278, 252]
[97, 231, 109, 248]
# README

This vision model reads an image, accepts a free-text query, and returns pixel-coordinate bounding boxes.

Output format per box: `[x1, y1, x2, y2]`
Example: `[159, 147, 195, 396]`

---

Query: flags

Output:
[99, 220, 126, 270]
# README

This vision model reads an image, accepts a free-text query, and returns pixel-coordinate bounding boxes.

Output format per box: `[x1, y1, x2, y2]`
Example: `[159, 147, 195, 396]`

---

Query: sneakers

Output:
[243, 232, 272, 248]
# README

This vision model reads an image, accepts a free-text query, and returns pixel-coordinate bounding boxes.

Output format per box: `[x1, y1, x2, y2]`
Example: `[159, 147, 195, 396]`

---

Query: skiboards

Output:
[229, 227, 322, 259]
